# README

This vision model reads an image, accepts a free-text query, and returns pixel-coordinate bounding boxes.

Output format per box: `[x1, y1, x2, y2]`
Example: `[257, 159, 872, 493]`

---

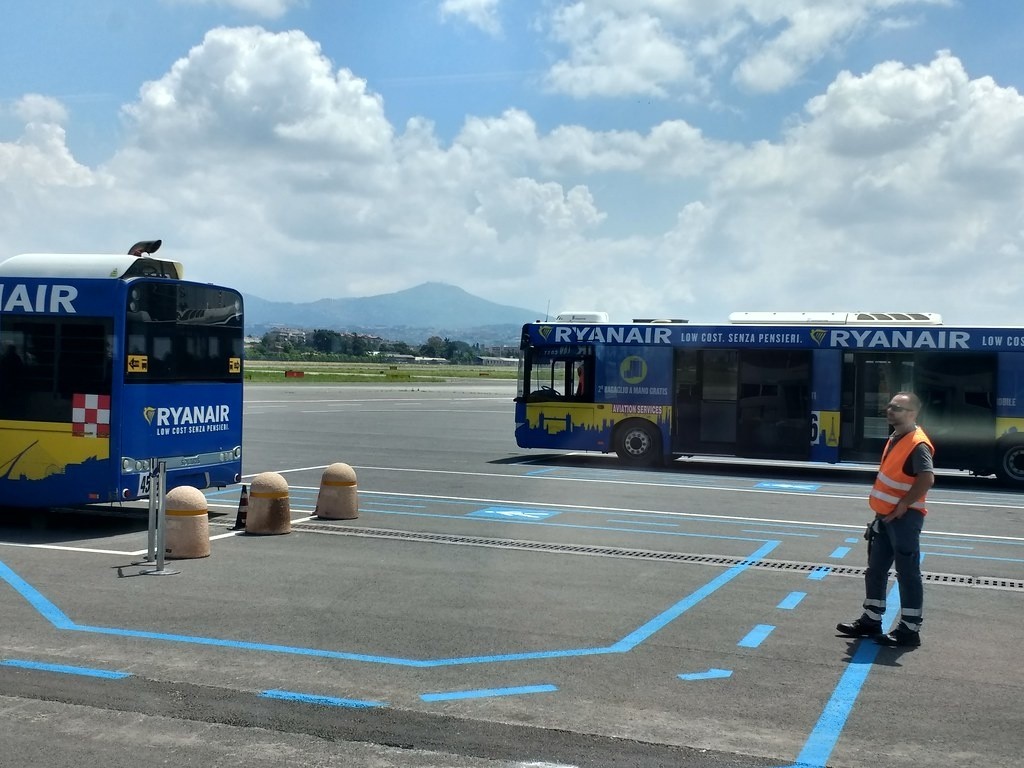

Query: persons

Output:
[836, 390, 935, 647]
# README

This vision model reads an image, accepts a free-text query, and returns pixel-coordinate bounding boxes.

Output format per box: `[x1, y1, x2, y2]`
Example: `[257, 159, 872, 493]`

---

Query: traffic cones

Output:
[227, 484, 250, 532]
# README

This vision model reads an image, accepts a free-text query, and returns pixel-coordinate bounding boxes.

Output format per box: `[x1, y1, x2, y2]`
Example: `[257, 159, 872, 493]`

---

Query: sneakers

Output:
[835, 612, 883, 638]
[870, 628, 922, 646]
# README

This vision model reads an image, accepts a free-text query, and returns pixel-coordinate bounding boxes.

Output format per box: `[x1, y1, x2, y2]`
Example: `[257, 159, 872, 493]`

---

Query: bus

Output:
[0, 239, 244, 517]
[514, 300, 1024, 490]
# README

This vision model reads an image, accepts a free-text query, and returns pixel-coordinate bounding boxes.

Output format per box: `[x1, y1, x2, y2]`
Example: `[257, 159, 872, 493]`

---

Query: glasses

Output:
[887, 401, 913, 413]
